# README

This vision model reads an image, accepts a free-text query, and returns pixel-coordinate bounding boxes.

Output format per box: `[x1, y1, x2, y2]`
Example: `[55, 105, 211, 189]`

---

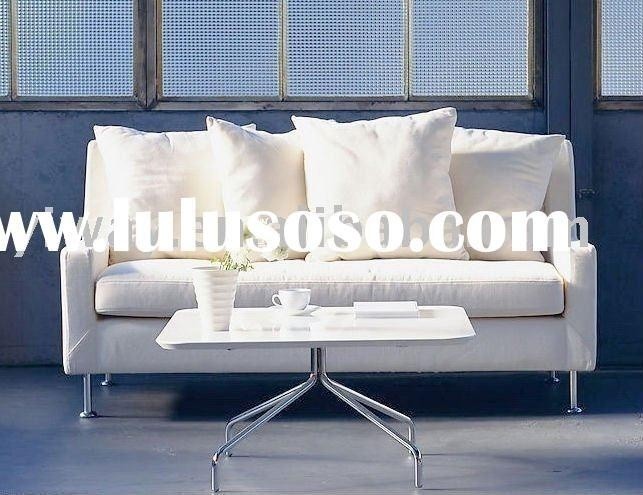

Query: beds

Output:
[157, 306, 478, 495]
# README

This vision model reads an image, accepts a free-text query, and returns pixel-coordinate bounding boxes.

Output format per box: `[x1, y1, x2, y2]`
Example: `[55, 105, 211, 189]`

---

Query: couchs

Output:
[60, 139, 596, 419]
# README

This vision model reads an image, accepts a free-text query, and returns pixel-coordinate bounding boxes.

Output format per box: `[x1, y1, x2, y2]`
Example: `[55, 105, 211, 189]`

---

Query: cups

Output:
[270, 288, 311, 312]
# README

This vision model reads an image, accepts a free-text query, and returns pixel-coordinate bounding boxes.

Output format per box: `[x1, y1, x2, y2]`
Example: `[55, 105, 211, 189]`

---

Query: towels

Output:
[353, 301, 419, 319]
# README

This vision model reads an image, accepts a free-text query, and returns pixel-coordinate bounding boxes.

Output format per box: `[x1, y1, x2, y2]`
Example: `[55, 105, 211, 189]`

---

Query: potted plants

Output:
[190, 227, 287, 331]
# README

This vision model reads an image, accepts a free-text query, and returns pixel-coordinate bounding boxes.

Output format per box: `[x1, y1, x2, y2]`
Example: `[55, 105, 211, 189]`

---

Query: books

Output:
[352, 298, 420, 318]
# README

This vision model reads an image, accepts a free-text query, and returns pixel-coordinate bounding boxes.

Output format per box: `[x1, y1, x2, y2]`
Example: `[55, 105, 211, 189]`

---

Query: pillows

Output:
[205, 115, 308, 264]
[291, 106, 471, 261]
[447, 124, 566, 263]
[93, 122, 260, 265]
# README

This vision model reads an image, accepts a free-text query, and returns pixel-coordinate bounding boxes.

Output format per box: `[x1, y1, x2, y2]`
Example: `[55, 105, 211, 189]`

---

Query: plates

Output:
[266, 304, 320, 316]
